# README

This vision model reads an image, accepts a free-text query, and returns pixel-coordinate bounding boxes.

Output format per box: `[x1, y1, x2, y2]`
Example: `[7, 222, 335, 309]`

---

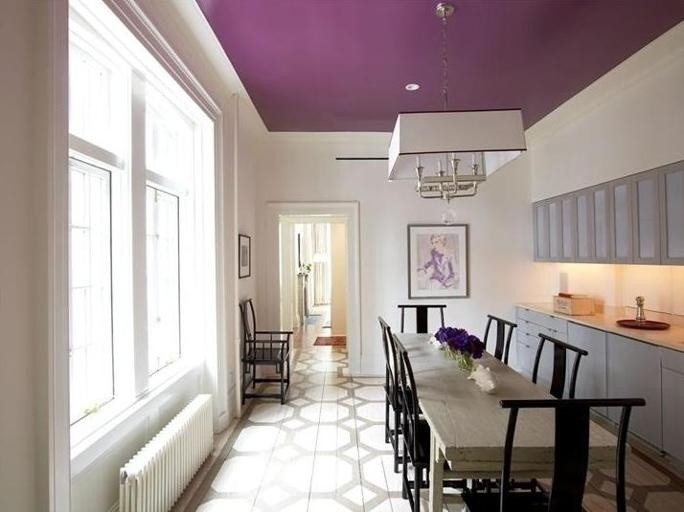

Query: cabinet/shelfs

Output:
[531, 160, 684, 265]
[515, 306, 683, 483]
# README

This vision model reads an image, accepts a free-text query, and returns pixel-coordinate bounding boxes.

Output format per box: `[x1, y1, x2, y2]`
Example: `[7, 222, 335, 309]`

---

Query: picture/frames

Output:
[238, 233, 251, 279]
[407, 222, 469, 300]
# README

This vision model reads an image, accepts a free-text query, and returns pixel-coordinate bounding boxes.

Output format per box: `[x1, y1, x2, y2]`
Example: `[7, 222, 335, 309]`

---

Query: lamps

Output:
[387, 4, 528, 225]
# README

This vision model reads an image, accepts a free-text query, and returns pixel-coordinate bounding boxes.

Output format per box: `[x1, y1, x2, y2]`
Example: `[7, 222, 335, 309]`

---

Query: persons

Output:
[415, 233, 456, 288]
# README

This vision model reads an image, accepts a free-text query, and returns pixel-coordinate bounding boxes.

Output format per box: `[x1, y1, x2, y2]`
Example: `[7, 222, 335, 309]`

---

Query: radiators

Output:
[119, 393, 215, 512]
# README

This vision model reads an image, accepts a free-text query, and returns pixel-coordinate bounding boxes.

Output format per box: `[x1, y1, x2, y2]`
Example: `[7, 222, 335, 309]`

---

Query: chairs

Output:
[238, 297, 294, 405]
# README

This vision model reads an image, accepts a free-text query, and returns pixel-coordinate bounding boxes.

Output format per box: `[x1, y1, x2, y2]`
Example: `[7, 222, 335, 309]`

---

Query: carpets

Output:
[313, 336, 346, 347]
